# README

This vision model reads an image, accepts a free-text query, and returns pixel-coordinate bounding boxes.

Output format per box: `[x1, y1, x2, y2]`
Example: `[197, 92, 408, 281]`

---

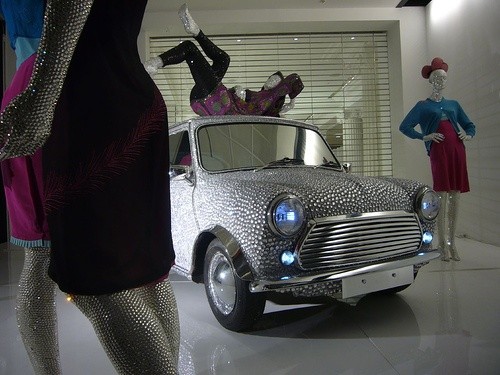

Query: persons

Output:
[0, 1, 71, 374]
[398, 56, 476, 262]
[141, 2, 305, 118]
[1, 1, 181, 374]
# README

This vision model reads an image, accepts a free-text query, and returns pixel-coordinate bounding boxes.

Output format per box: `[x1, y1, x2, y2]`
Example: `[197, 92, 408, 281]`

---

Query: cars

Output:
[168, 113, 443, 332]
